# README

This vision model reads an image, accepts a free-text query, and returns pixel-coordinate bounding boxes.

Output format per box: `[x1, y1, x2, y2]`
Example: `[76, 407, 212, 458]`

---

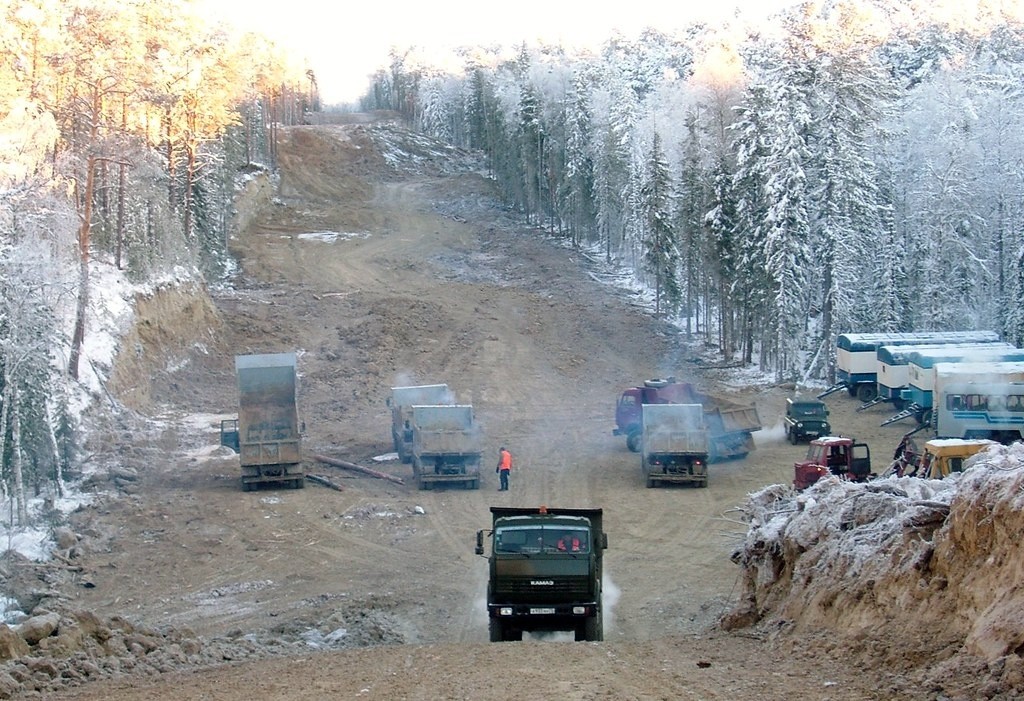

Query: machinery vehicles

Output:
[793, 435, 879, 490]
[915, 439, 999, 479]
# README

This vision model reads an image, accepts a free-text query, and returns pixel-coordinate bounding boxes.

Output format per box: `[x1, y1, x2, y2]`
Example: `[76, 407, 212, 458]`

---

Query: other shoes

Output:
[498, 488, 505, 491]
[505, 488, 508, 490]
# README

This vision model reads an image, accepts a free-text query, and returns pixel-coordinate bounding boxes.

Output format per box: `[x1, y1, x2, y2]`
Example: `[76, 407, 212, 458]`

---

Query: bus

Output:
[877, 342, 1017, 409]
[931, 361, 1024, 448]
[835, 330, 1005, 401]
[908, 348, 1024, 424]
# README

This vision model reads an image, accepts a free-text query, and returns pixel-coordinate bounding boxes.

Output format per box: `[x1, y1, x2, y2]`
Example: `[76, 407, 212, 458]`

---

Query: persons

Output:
[558, 531, 579, 551]
[496, 447, 513, 492]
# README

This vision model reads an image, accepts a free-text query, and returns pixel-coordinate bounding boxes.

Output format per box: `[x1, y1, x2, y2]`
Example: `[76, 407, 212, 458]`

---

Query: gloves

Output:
[508, 471, 510, 475]
[496, 467, 499, 473]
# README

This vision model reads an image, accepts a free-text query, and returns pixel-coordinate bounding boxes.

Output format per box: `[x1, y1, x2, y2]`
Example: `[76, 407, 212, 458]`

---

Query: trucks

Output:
[612, 378, 762, 465]
[783, 398, 831, 445]
[641, 403, 709, 488]
[384, 383, 455, 464]
[475, 505, 609, 643]
[220, 353, 308, 492]
[404, 404, 481, 491]
